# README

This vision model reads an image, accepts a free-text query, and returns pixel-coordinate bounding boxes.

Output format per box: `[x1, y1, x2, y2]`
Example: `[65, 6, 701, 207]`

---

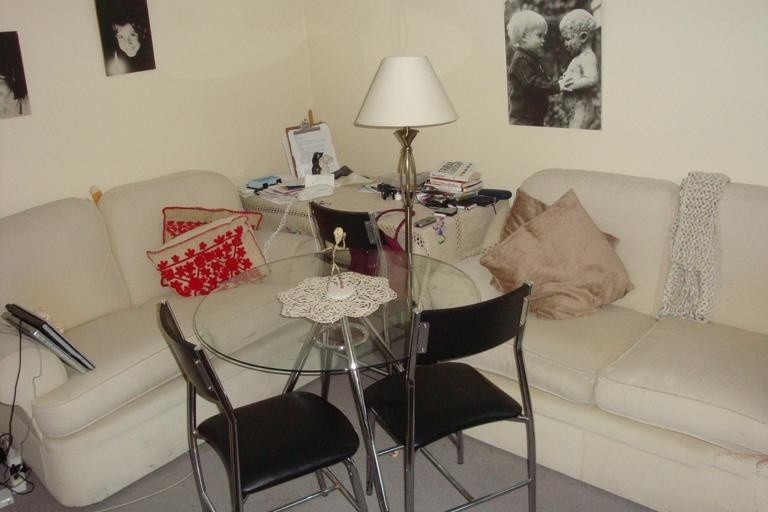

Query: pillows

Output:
[160, 206, 263, 287]
[146, 213, 270, 298]
[489, 190, 621, 292]
[479, 188, 636, 318]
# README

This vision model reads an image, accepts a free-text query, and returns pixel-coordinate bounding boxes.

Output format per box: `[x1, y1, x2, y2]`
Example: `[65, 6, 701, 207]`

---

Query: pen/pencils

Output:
[364, 185, 380, 195]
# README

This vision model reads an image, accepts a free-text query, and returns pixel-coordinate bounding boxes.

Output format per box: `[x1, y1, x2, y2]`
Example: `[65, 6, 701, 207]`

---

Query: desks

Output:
[239, 172, 510, 269]
[194, 250, 478, 512]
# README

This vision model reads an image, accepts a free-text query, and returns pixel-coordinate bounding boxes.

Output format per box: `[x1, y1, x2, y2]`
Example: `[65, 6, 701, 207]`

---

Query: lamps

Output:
[354, 56, 460, 365]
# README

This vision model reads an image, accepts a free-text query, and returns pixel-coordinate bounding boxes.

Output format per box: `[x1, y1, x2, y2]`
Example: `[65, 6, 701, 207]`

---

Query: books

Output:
[421, 159, 484, 202]
[255, 180, 304, 206]
[4, 303, 97, 371]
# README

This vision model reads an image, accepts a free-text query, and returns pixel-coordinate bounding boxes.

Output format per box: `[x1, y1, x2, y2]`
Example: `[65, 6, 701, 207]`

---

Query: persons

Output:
[108, 13, 148, 61]
[0, 66, 31, 117]
[556, 7, 599, 128]
[504, 9, 573, 126]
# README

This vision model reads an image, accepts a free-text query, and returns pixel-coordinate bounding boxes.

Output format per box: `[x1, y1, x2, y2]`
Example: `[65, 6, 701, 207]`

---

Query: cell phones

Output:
[414, 216, 436, 228]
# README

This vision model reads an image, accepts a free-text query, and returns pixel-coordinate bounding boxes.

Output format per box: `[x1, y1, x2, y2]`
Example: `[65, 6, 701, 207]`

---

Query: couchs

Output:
[414, 171, 768, 512]
[0, 169, 326, 512]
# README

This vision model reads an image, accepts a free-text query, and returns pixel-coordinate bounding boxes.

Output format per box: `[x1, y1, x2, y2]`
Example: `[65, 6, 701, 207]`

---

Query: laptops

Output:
[2, 312, 89, 374]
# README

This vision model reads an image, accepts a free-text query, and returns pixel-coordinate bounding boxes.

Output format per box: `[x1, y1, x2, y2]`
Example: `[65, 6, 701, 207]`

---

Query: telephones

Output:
[298, 184, 334, 201]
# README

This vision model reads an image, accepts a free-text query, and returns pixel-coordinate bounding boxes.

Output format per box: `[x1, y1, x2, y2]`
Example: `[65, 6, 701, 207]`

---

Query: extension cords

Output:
[0, 438, 24, 468]
[9, 465, 28, 493]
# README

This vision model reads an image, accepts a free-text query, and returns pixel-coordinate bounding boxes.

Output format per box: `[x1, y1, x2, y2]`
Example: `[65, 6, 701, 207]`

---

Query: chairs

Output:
[157, 303, 372, 512]
[307, 199, 408, 377]
[366, 281, 538, 512]
